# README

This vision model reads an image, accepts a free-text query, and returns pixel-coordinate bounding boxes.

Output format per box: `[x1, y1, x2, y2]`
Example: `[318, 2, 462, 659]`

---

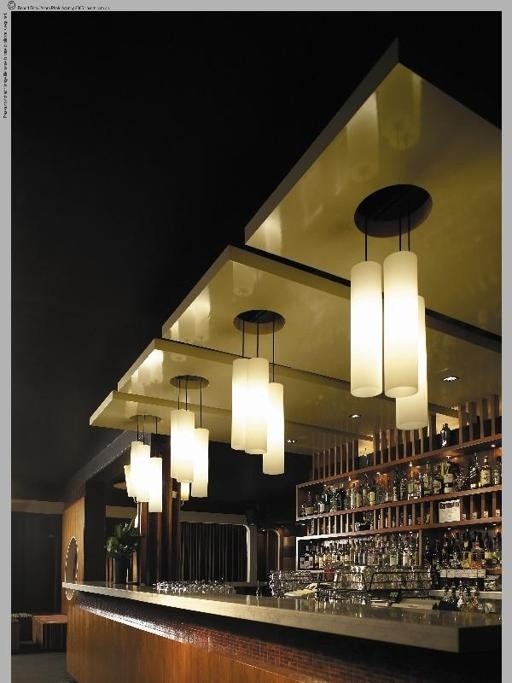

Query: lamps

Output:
[349, 184, 429, 431]
[124, 415, 162, 512]
[171, 375, 209, 500]
[232, 310, 284, 475]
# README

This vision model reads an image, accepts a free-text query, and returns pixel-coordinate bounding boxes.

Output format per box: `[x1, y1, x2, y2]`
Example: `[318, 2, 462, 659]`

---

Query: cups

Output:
[156, 580, 234, 595]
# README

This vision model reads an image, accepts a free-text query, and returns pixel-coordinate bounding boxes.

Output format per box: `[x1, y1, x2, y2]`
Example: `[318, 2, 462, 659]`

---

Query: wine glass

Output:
[441, 586, 479, 613]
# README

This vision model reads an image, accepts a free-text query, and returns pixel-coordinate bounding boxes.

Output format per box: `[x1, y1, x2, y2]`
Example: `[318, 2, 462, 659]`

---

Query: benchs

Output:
[31, 613, 67, 653]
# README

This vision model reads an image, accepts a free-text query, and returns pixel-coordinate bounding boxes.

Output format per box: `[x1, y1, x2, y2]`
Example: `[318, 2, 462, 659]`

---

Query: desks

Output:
[11, 612, 32, 653]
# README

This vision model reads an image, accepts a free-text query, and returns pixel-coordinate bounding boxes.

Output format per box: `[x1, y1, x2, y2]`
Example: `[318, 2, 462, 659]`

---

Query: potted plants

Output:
[104, 513, 141, 584]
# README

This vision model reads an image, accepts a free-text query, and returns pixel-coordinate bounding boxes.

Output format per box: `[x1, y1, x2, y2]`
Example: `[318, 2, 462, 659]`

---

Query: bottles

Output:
[299, 479, 385, 517]
[385, 452, 502, 505]
[424, 532, 502, 570]
[299, 532, 418, 569]
[440, 423, 451, 447]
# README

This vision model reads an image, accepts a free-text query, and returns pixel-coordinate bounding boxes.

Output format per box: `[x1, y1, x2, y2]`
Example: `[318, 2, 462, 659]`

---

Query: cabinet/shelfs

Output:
[295, 432, 502, 593]
